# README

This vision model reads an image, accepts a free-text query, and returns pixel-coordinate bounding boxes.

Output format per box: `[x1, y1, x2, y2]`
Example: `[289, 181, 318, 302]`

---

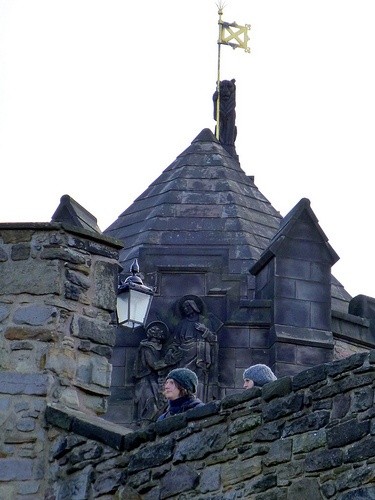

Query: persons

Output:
[156, 367, 206, 423]
[242, 364, 279, 390]
[129, 320, 184, 429]
[162, 294, 220, 405]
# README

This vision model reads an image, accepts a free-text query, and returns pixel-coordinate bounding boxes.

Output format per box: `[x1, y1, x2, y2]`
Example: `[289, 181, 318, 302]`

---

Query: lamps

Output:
[112, 257, 157, 336]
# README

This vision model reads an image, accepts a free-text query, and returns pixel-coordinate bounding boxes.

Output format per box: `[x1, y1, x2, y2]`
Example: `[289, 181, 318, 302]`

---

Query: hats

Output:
[165, 368, 198, 393]
[244, 363, 277, 385]
